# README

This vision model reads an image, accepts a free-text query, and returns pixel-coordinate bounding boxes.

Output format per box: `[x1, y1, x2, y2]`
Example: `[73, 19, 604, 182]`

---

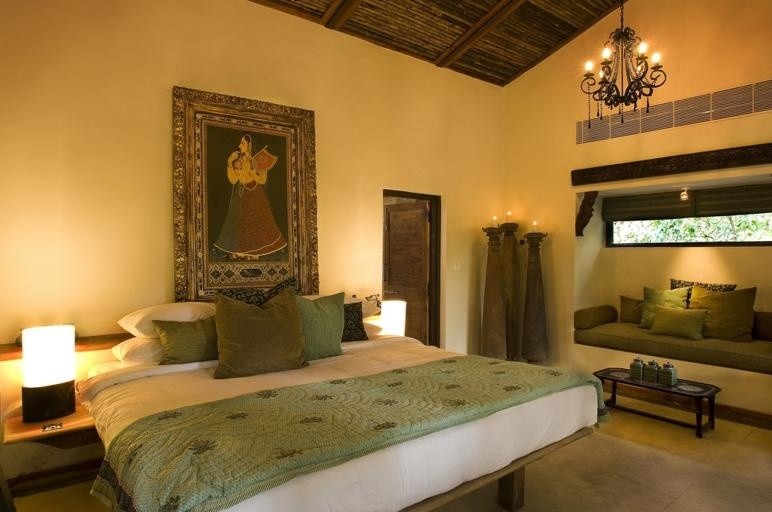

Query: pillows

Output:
[574, 278, 772, 343]
[110, 275, 383, 379]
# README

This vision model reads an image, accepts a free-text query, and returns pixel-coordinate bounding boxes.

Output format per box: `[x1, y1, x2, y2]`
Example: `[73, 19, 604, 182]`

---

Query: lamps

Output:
[380, 300, 407, 337]
[580, 0, 666, 129]
[20, 323, 77, 425]
[680, 187, 690, 201]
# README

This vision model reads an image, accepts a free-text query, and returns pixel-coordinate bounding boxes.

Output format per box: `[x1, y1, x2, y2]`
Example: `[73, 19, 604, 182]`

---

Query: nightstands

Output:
[0, 404, 107, 498]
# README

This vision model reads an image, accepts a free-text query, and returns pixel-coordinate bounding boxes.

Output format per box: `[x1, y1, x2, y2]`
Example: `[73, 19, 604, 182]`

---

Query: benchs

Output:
[574, 322, 772, 418]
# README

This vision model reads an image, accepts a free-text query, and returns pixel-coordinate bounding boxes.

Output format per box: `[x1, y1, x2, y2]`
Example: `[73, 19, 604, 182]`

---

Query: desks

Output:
[593, 367, 722, 439]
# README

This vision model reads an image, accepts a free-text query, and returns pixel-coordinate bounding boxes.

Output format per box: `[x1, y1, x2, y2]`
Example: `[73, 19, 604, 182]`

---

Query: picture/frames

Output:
[171, 84, 320, 303]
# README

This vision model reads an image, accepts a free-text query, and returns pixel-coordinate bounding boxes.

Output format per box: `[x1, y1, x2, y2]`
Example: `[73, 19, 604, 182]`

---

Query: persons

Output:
[213, 132, 288, 262]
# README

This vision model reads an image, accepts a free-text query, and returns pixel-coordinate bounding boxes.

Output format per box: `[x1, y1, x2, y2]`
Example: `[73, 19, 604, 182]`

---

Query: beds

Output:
[78, 334, 609, 512]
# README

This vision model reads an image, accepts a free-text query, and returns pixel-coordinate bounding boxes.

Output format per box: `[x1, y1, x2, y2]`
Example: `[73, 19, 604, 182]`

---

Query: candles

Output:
[507, 212, 513, 223]
[531, 221, 538, 232]
[491, 216, 499, 226]
